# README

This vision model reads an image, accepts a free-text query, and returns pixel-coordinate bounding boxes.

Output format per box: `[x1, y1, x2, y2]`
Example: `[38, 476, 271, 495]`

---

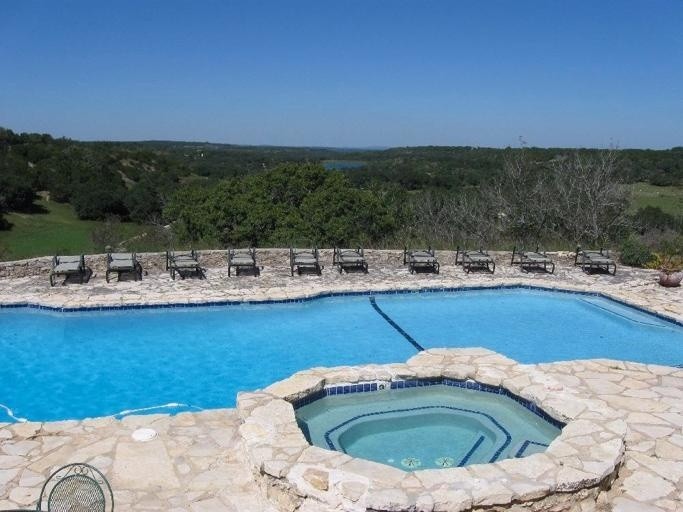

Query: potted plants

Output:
[643, 251, 683, 287]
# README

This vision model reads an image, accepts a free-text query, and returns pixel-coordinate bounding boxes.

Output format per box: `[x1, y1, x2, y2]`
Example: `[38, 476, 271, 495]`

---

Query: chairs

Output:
[333, 245, 368, 274]
[165, 249, 201, 280]
[403, 245, 439, 275]
[289, 246, 321, 276]
[575, 245, 616, 276]
[37, 462, 115, 512]
[105, 250, 138, 282]
[49, 253, 85, 286]
[454, 245, 495, 275]
[509, 242, 555, 275]
[226, 246, 258, 279]
